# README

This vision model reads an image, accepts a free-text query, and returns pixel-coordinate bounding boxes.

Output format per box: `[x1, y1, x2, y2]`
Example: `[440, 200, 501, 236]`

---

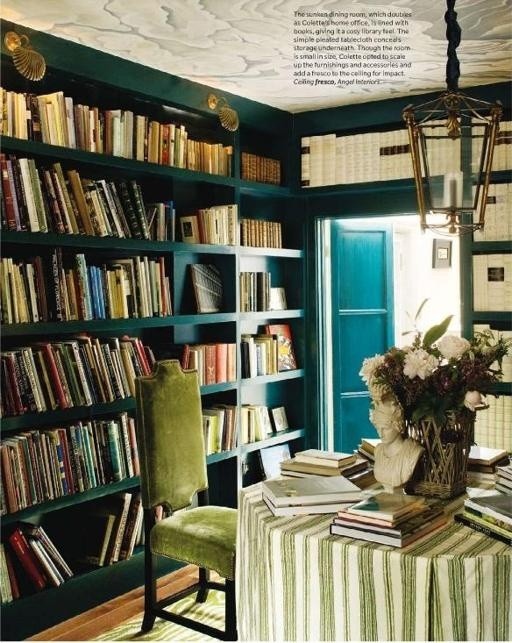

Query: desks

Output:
[235, 473, 512, 642]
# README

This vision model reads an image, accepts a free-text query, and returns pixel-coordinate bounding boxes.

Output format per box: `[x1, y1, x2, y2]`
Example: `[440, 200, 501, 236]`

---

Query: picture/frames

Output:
[432, 239, 452, 268]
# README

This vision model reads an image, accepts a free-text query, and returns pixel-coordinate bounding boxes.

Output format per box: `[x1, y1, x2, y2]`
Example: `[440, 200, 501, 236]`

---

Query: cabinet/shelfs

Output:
[460, 81, 512, 455]
[0, 17, 308, 641]
[294, 81, 512, 197]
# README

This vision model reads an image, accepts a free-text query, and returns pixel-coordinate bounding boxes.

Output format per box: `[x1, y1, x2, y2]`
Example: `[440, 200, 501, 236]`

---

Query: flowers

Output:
[357, 301, 512, 429]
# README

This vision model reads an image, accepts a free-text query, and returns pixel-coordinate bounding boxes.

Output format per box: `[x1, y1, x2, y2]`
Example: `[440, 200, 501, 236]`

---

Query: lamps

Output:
[402, 0, 503, 237]
[3, 31, 46, 82]
[207, 92, 239, 131]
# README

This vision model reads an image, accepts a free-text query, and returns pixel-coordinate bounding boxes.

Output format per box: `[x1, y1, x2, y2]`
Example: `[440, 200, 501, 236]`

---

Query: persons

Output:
[2, 85, 511, 605]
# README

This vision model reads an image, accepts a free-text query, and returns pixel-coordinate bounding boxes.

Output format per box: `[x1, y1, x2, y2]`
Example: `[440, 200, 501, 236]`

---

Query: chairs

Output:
[134, 359, 237, 642]
